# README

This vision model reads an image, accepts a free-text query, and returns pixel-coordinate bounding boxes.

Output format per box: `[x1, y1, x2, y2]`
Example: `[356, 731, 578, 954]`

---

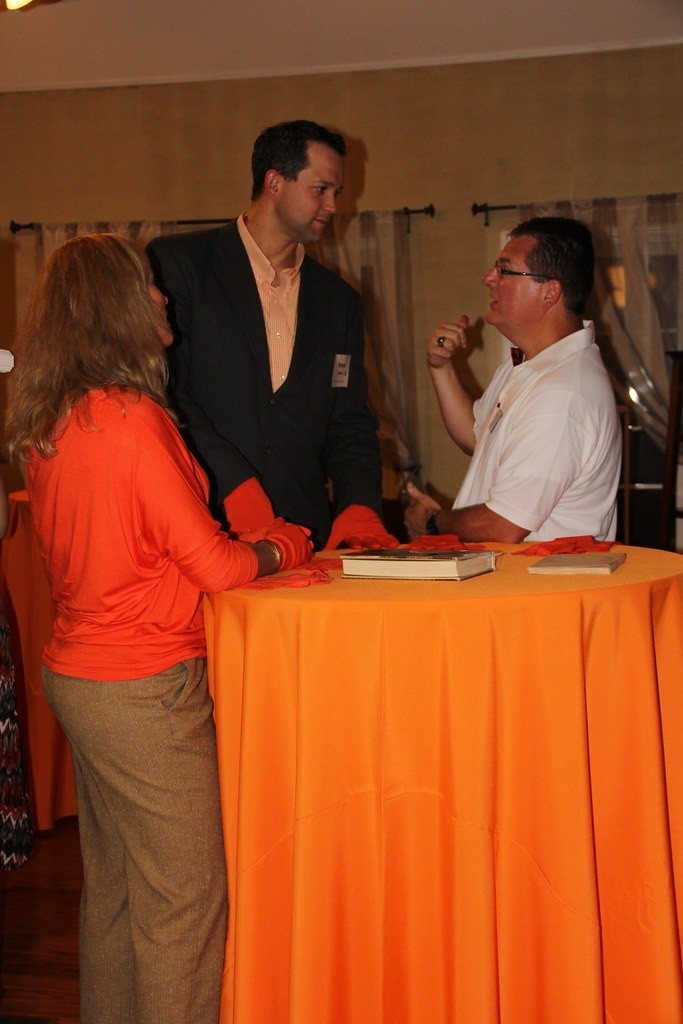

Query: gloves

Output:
[404, 534, 466, 551]
[512, 534, 601, 557]
[238, 517, 314, 549]
[321, 504, 400, 550]
[223, 478, 274, 535]
[264, 516, 312, 573]
[572, 540, 622, 553]
[238, 568, 332, 592]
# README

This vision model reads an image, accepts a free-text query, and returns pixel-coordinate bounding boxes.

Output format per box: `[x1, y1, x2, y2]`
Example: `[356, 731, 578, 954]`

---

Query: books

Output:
[527, 552, 627, 575]
[339, 547, 496, 581]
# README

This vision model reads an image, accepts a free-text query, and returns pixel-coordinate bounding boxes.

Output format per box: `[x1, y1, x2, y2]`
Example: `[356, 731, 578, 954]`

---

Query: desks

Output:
[0, 489, 80, 829]
[204, 542, 683, 1024]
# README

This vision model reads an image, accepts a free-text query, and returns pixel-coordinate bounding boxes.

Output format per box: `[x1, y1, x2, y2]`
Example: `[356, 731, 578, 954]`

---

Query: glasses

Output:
[495, 260, 549, 279]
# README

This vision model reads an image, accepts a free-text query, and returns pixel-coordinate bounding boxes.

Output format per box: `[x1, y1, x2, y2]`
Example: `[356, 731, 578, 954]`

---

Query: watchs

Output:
[426, 510, 441, 535]
[263, 540, 282, 574]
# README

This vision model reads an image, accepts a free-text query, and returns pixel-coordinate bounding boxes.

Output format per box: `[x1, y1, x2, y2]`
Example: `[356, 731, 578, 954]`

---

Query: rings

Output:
[437, 336, 446, 347]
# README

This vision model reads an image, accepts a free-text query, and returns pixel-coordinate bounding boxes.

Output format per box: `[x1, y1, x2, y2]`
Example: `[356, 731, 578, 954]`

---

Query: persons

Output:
[404, 217, 622, 544]
[6, 234, 315, 1024]
[145, 120, 401, 552]
